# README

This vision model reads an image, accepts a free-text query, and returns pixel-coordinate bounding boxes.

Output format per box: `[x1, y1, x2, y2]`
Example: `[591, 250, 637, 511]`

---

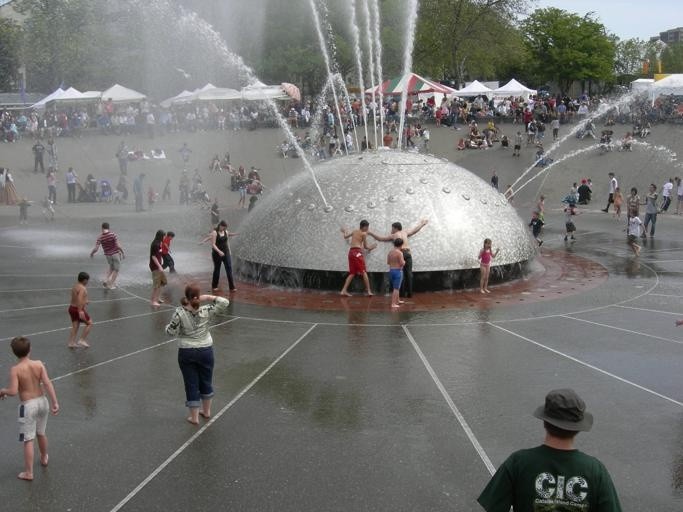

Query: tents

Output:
[650, 73, 683, 106]
[364, 72, 458, 118]
[632, 79, 654, 91]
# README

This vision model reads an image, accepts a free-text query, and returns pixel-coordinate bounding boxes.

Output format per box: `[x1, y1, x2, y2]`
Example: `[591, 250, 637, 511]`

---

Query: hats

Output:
[534, 387, 594, 431]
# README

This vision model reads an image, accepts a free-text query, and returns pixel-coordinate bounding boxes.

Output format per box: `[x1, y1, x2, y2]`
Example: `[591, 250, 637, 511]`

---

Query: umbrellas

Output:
[281, 82, 300, 101]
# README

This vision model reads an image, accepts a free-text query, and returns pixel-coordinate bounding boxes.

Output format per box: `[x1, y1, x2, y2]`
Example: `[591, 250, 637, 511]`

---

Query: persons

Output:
[478, 239, 500, 293]
[67, 271, 93, 348]
[387, 238, 406, 308]
[339, 220, 378, 297]
[368, 219, 429, 297]
[1, 90, 683, 257]
[149, 230, 169, 306]
[477, 387, 623, 511]
[211, 220, 237, 292]
[160, 232, 175, 273]
[0, 337, 59, 480]
[91, 223, 124, 290]
[166, 284, 230, 424]
